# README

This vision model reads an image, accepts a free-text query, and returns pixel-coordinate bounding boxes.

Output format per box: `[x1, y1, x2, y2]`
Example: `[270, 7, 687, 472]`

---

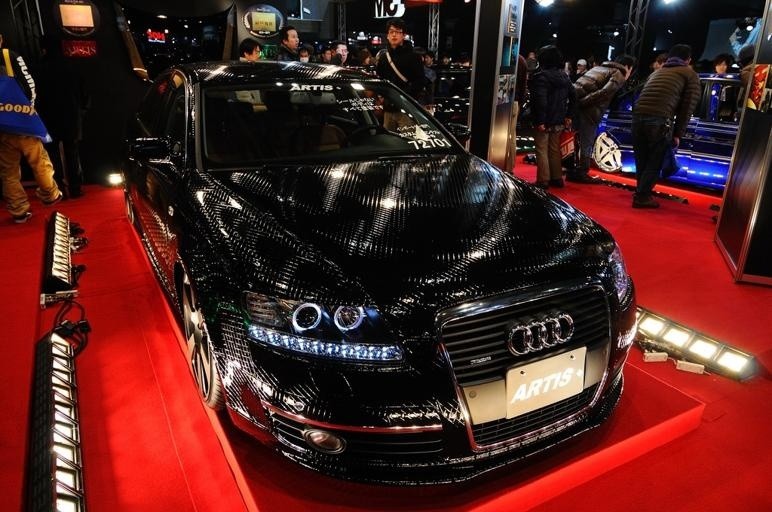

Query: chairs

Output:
[204, 90, 349, 164]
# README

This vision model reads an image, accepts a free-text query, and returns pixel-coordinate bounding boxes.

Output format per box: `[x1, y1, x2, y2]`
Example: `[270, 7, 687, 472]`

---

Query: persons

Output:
[738, 44, 756, 69]
[514, 51, 733, 129]
[376, 18, 425, 137]
[529, 43, 579, 190]
[235, 38, 261, 102]
[632, 42, 704, 209]
[566, 52, 637, 182]
[274, 26, 349, 66]
[353, 44, 437, 115]
[0, 30, 63, 226]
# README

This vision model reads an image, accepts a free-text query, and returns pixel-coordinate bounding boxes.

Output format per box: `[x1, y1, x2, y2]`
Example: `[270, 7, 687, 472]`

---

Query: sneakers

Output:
[532, 170, 604, 191]
[631, 194, 660, 209]
[13, 206, 34, 224]
[41, 189, 64, 208]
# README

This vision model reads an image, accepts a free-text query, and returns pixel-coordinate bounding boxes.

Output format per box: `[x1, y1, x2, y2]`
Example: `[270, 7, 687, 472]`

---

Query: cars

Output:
[120, 59, 637, 487]
[590, 72, 743, 191]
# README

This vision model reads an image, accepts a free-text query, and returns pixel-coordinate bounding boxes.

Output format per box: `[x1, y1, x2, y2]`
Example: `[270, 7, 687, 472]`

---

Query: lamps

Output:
[632, 306, 759, 382]
[39, 211, 89, 308]
[26, 319, 91, 512]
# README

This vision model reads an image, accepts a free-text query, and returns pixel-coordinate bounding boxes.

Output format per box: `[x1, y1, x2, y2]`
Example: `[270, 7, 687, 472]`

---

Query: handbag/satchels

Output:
[653, 133, 682, 183]
[0, 72, 50, 140]
